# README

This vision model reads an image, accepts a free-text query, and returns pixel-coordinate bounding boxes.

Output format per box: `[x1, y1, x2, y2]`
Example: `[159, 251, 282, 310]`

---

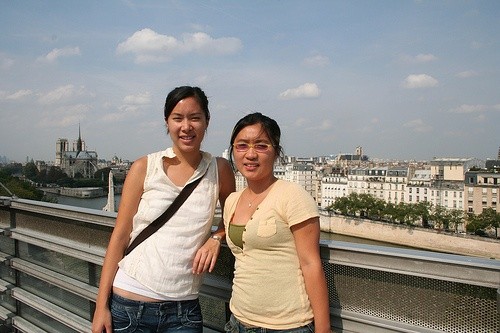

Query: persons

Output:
[223, 112, 335, 333]
[92, 85, 236, 333]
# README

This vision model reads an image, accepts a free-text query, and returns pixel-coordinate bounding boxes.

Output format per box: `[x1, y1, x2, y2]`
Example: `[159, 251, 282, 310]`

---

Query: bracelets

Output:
[209, 235, 222, 242]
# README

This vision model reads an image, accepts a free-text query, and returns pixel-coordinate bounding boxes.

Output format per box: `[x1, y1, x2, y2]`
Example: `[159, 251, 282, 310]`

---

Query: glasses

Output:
[232, 142, 277, 152]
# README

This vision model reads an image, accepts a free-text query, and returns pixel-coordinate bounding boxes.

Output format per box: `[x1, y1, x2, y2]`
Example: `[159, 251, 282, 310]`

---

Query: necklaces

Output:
[248, 185, 271, 207]
